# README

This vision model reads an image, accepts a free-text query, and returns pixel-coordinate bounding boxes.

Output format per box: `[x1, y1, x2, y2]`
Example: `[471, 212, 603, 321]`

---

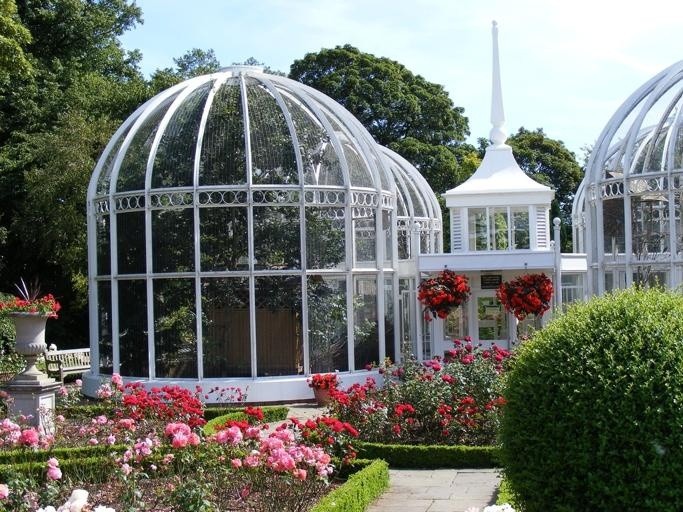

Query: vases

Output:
[5, 311, 53, 374]
[312, 387, 334, 405]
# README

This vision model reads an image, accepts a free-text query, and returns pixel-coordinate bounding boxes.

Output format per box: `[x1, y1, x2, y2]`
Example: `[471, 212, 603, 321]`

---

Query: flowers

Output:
[492, 272, 553, 320]
[306, 373, 341, 392]
[0, 292, 60, 320]
[413, 265, 471, 322]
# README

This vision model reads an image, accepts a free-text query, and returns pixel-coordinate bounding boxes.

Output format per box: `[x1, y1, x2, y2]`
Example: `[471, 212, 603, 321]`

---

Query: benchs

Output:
[41, 347, 90, 383]
